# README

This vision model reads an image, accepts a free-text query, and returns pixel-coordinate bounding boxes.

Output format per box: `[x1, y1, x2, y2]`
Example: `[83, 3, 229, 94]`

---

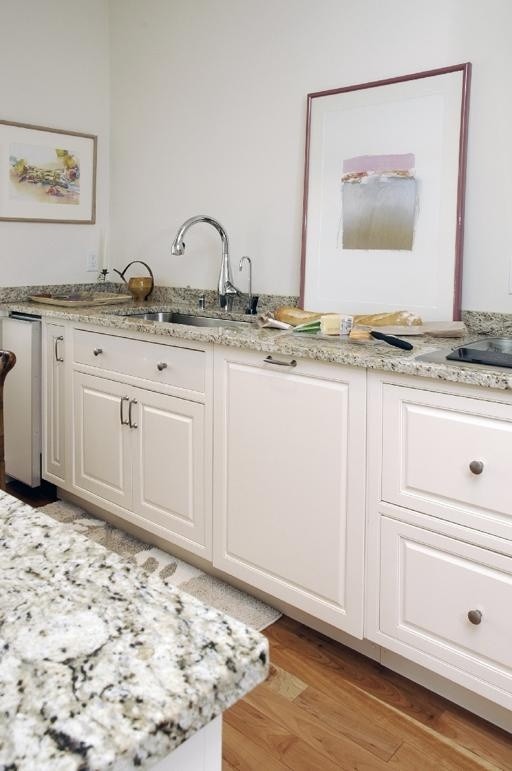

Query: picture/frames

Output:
[0, 119, 97, 226]
[298, 62, 472, 323]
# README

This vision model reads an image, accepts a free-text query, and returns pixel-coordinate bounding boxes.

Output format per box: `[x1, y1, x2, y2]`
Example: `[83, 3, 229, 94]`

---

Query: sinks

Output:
[121, 312, 255, 329]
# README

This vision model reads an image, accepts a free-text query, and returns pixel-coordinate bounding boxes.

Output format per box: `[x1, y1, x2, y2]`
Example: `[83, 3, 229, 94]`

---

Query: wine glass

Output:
[128, 277, 153, 305]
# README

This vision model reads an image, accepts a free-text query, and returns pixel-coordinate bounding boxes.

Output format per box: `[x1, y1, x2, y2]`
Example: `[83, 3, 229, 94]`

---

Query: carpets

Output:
[36, 499, 285, 633]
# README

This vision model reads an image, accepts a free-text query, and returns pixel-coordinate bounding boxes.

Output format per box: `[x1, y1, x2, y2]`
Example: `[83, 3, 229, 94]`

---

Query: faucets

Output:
[239, 256, 251, 308]
[171, 215, 232, 296]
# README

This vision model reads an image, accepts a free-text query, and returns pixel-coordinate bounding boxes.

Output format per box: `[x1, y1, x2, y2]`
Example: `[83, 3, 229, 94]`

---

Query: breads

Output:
[275, 304, 422, 327]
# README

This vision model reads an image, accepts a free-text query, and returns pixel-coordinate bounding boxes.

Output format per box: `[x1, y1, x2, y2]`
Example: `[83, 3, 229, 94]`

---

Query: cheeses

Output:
[318, 313, 353, 336]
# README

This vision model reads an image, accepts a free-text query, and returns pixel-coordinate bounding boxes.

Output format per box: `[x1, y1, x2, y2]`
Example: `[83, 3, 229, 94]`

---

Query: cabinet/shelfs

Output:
[68, 321, 213, 577]
[364, 369, 512, 734]
[39, 317, 69, 505]
[213, 344, 367, 657]
